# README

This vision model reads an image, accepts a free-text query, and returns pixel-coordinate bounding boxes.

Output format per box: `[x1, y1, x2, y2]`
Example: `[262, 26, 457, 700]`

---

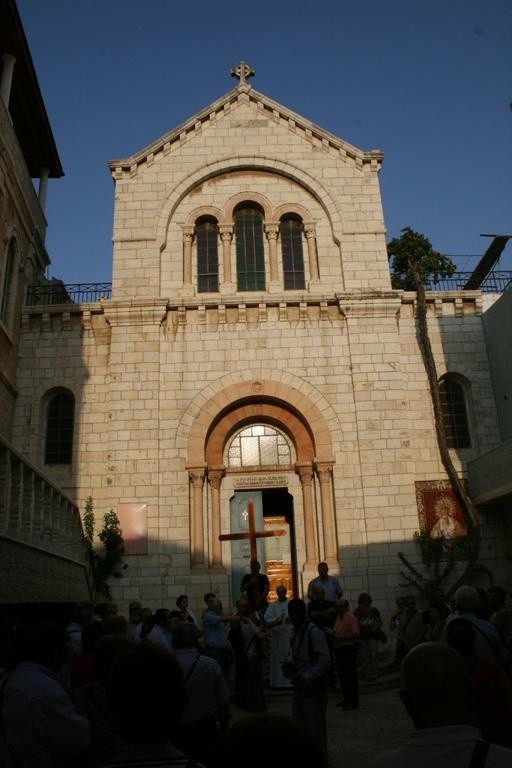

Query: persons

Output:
[389, 586, 511, 768]
[429, 497, 466, 539]
[307, 562, 343, 603]
[326, 593, 383, 713]
[2, 585, 333, 768]
[240, 560, 270, 612]
